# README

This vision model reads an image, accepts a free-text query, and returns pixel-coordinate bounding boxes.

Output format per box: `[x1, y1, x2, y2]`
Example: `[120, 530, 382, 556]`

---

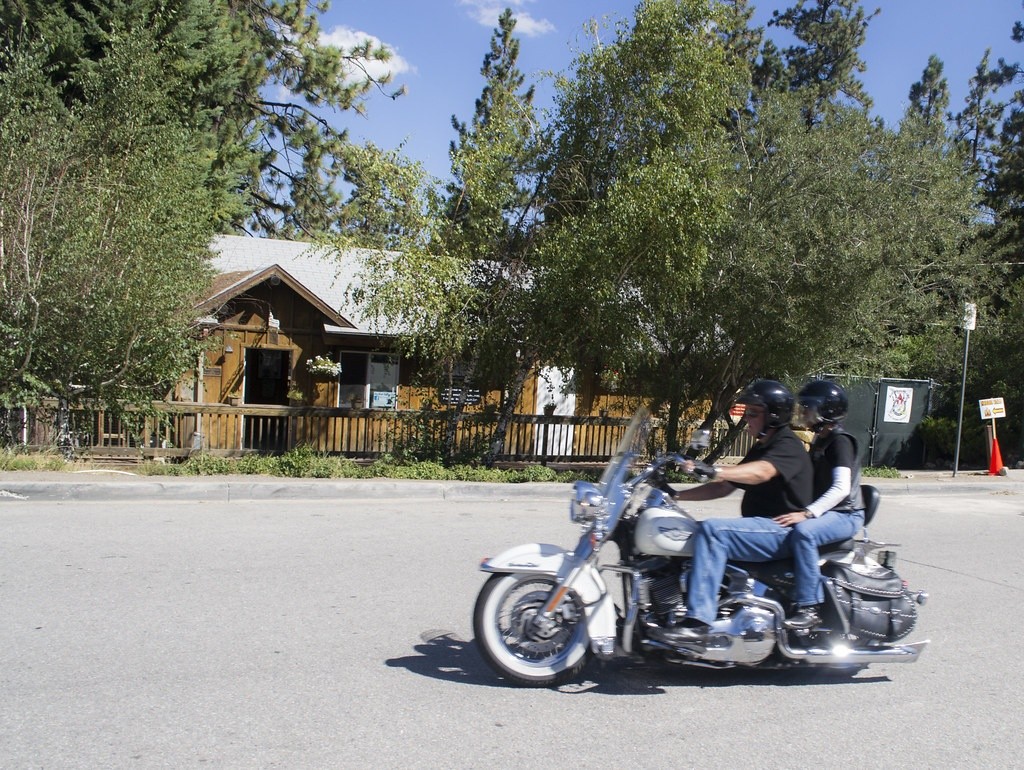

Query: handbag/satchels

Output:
[821, 548, 917, 641]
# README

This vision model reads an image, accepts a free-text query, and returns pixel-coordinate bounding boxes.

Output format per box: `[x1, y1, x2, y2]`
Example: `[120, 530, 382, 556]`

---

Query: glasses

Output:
[744, 408, 765, 419]
[800, 400, 822, 408]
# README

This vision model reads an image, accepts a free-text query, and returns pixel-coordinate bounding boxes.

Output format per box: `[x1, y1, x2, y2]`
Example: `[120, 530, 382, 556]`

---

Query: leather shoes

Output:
[644, 626, 714, 654]
[781, 604, 825, 629]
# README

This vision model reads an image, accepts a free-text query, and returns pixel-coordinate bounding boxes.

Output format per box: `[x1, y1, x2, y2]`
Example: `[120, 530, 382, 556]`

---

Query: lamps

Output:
[225, 345, 234, 353]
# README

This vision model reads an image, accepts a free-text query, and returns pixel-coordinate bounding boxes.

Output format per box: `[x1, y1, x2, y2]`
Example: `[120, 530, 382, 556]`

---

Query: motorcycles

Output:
[473, 406, 933, 687]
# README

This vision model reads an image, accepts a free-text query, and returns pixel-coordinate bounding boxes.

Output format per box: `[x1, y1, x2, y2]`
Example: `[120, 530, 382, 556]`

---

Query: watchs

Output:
[805, 509, 814, 520]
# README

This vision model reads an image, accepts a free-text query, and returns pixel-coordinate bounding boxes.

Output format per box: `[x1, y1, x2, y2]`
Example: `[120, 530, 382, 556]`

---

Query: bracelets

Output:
[708, 467, 723, 483]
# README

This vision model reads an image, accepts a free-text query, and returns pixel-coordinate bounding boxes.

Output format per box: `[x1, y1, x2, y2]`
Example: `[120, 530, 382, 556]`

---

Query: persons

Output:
[648, 379, 812, 647]
[772, 381, 865, 630]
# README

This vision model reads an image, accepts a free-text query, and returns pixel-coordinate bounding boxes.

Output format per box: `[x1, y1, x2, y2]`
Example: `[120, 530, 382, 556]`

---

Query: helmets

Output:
[798, 381, 848, 424]
[734, 380, 794, 428]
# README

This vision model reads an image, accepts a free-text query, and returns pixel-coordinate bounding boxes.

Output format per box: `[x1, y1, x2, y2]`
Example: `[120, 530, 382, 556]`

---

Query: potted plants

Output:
[420, 399, 433, 410]
[599, 405, 609, 416]
[287, 389, 306, 407]
[228, 392, 240, 406]
[544, 403, 557, 415]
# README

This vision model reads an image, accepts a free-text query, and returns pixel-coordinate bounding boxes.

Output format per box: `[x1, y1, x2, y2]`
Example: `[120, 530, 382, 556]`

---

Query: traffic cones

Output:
[986, 437, 1004, 475]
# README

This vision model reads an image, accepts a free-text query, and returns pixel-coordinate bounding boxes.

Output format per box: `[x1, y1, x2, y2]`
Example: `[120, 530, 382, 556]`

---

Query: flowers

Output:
[306, 354, 342, 378]
